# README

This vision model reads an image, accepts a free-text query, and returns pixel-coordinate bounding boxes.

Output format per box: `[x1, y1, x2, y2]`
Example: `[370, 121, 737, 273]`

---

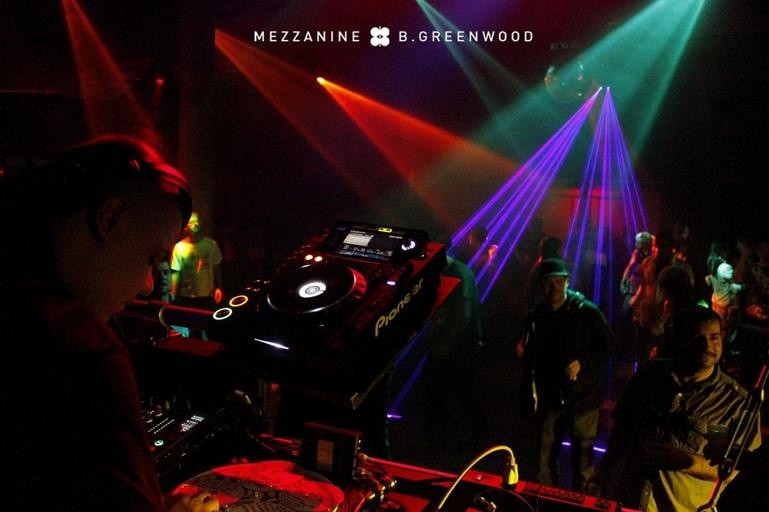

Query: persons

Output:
[146, 257, 187, 305]
[171, 210, 223, 309]
[0, 133, 221, 511]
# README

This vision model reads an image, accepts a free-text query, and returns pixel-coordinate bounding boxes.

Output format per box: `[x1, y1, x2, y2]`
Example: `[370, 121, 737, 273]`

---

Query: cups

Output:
[707, 425, 729, 440]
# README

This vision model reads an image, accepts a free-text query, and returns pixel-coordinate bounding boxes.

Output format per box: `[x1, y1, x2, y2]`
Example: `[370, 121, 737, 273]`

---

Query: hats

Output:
[539, 257, 569, 279]
